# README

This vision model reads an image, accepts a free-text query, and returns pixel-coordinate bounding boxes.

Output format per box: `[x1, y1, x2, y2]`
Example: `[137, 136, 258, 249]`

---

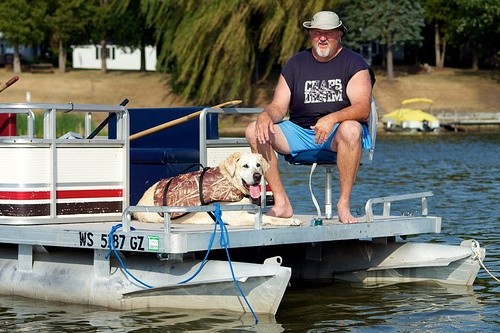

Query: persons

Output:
[246, 11, 377, 223]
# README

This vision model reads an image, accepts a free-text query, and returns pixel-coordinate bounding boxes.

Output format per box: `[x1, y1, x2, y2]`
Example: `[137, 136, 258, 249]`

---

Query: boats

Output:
[0, 100, 487, 316]
[382, 98, 439, 134]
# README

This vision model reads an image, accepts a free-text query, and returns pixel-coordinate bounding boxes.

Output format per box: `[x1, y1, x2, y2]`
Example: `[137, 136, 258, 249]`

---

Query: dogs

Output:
[133, 152, 302, 226]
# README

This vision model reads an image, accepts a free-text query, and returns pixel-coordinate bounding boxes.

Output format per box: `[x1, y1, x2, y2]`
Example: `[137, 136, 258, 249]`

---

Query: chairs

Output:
[285, 100, 377, 223]
